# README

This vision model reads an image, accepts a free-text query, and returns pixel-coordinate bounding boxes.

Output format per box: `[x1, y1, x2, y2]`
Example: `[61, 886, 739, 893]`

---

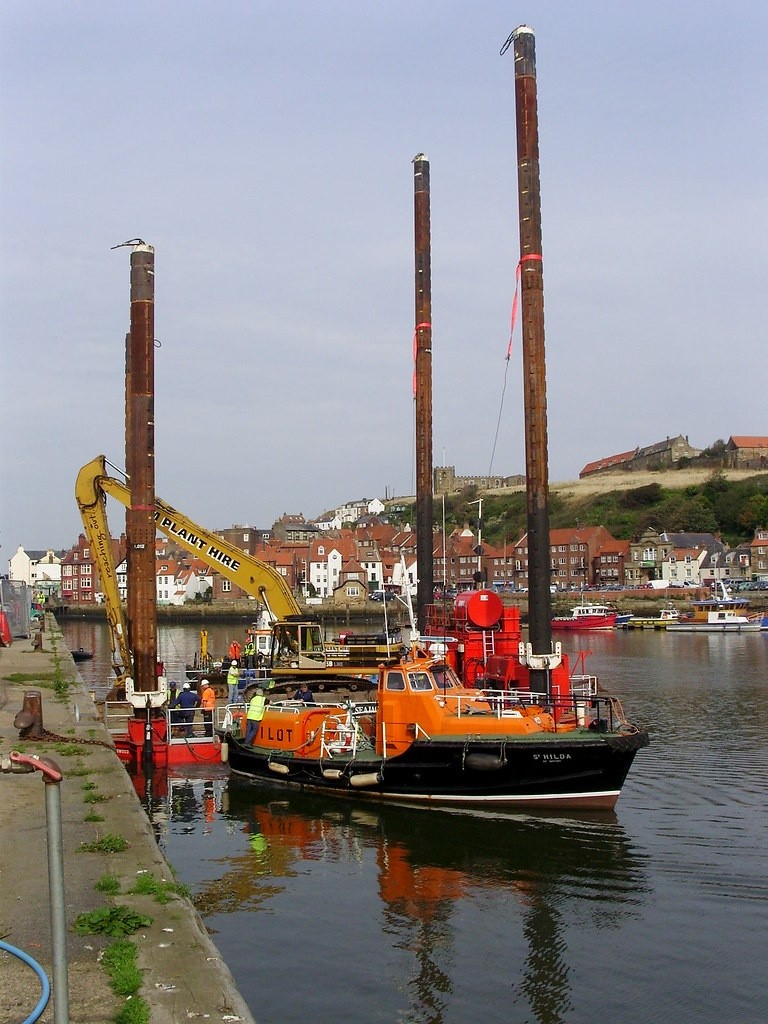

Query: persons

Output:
[156, 657, 164, 677]
[243, 688, 273, 747]
[239, 670, 255, 685]
[432, 582, 470, 600]
[229, 639, 242, 668]
[281, 630, 298, 653]
[227, 660, 239, 704]
[244, 637, 254, 668]
[49, 553, 53, 564]
[165, 679, 215, 738]
[31, 590, 45, 611]
[294, 683, 317, 707]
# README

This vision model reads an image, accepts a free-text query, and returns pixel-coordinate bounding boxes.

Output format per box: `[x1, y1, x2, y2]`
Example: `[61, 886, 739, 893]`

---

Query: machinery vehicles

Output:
[72, 454, 407, 710]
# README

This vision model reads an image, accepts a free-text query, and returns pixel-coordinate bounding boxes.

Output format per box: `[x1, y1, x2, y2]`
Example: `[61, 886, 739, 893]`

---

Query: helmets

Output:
[200, 680, 209, 687]
[256, 689, 263, 695]
[231, 660, 237, 665]
[182, 683, 190, 688]
[247, 638, 251, 641]
[170, 682, 176, 687]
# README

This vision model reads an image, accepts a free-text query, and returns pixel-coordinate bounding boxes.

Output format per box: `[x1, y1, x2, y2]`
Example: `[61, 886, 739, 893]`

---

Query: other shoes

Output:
[244, 743, 253, 749]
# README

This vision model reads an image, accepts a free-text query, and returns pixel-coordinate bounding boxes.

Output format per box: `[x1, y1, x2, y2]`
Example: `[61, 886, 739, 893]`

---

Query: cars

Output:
[710, 579, 768, 592]
[368, 590, 396, 602]
[433, 587, 528, 600]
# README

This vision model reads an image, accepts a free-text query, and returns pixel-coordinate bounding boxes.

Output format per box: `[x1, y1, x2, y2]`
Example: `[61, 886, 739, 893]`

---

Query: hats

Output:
[300, 684, 307, 688]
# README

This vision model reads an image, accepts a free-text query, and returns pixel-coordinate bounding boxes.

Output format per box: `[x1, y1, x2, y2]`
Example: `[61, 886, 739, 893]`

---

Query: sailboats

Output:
[207, 25, 650, 811]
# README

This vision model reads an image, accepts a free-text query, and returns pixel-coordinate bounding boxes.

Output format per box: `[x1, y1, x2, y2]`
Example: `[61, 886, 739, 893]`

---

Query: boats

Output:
[104, 700, 221, 765]
[550, 578, 768, 631]
[71, 647, 93, 660]
